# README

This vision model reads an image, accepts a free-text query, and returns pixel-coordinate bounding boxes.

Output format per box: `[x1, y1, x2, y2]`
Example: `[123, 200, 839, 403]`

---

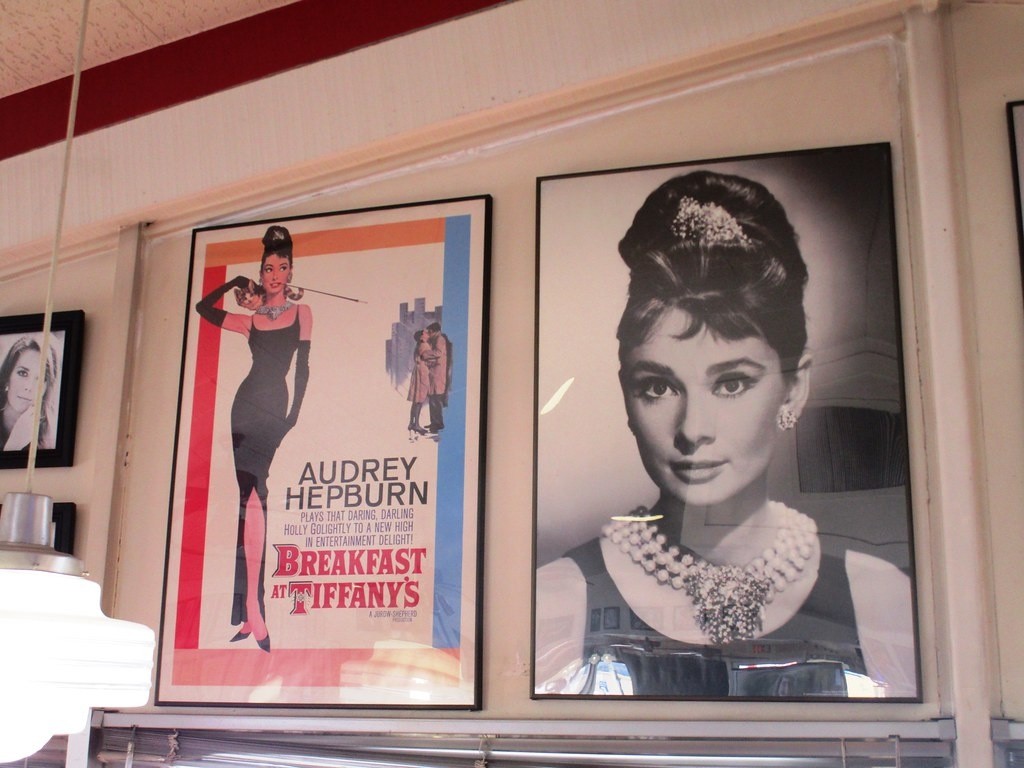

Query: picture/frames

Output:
[0, 310, 86, 468]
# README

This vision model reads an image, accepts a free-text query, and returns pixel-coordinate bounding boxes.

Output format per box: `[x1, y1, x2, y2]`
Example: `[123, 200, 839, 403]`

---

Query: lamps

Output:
[1, 0, 155, 764]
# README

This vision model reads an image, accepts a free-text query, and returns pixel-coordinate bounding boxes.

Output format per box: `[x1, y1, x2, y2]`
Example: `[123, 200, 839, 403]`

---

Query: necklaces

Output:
[601, 499, 816, 643]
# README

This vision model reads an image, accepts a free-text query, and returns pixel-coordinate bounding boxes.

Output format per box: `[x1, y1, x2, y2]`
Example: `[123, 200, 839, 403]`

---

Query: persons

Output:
[196, 226, 313, 651]
[0, 333, 58, 451]
[406, 323, 447, 441]
[535, 170, 916, 694]
